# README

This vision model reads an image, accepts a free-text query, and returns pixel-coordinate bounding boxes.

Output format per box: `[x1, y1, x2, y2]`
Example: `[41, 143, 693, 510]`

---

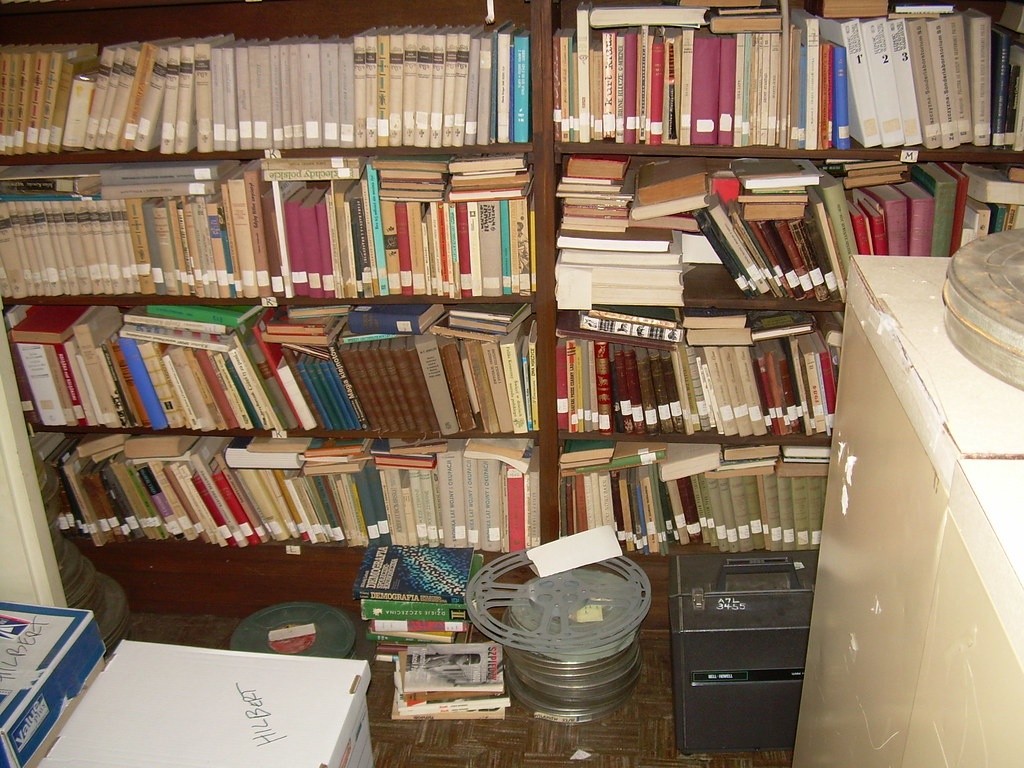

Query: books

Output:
[0, 0, 1024, 552]
[351, 544, 512, 720]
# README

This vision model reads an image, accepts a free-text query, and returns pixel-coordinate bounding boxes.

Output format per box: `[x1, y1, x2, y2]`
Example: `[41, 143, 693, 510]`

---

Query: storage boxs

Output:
[0, 597, 105, 768]
[38, 639, 377, 767]
[668, 550, 817, 754]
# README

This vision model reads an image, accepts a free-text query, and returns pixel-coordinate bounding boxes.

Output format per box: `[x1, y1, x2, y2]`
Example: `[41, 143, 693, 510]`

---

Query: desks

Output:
[793, 253, 1024, 768]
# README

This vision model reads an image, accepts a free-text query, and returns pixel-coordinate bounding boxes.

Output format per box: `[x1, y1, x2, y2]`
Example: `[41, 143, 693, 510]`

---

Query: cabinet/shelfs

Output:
[0, 0, 1024, 615]
